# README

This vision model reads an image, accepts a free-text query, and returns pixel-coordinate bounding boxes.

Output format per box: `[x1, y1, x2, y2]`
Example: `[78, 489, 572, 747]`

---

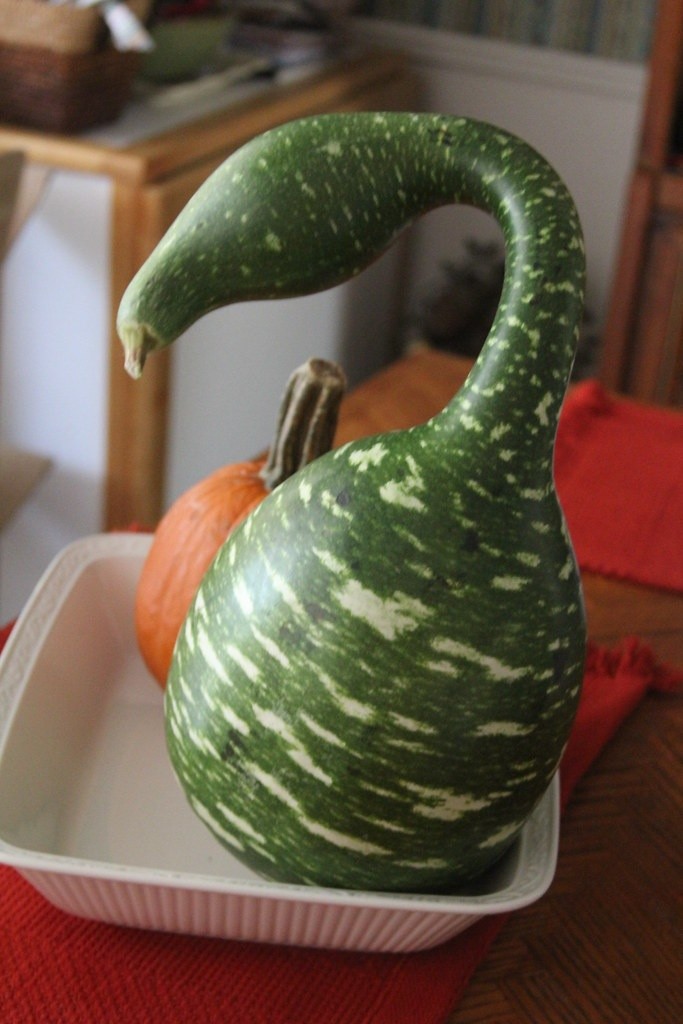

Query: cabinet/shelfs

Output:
[1, 52, 425, 624]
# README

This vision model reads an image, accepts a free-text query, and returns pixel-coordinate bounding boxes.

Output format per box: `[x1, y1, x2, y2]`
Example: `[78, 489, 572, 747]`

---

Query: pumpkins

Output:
[127, 356, 351, 690]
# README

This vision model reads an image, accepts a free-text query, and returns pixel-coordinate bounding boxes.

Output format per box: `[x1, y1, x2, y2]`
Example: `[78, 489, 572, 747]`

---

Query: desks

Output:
[0, 336, 683, 1024]
